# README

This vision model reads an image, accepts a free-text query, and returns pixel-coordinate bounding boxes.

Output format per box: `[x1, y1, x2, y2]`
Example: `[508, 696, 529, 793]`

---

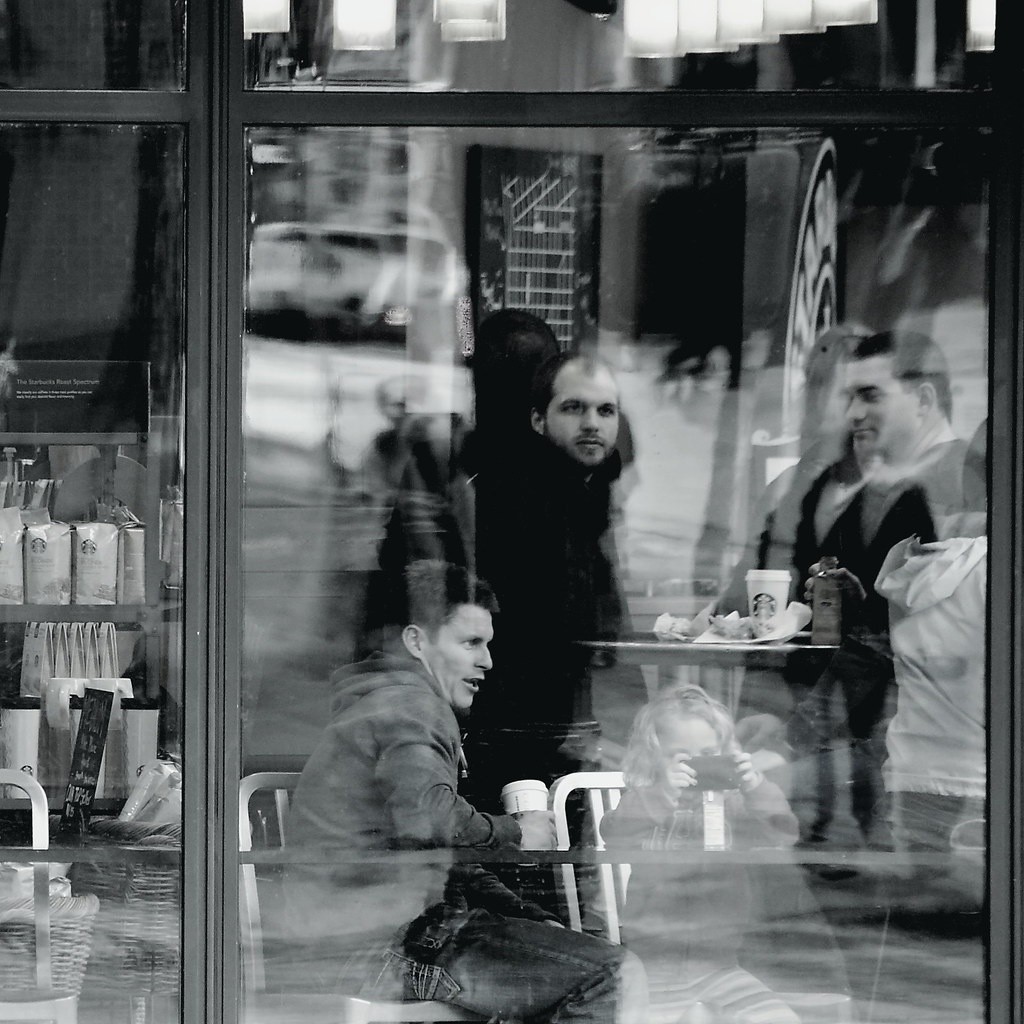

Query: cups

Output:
[743, 569, 793, 640]
[498, 778, 550, 867]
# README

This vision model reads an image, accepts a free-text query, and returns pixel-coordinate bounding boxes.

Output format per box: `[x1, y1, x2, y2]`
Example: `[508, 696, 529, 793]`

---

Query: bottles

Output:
[811, 555, 843, 646]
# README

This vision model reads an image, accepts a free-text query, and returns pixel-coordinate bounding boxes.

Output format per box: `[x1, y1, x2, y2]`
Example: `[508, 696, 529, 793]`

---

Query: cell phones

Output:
[683, 756, 740, 791]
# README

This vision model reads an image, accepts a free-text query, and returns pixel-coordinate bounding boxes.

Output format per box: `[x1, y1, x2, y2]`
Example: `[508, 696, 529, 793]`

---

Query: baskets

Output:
[49, 814, 183, 1024]
[0, 893, 100, 1001]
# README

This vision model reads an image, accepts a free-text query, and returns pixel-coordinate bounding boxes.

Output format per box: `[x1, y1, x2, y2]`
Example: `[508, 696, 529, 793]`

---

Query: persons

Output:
[873, 417, 988, 1024]
[283, 558, 645, 1024]
[324, 310, 989, 1023]
[599, 686, 801, 1024]
